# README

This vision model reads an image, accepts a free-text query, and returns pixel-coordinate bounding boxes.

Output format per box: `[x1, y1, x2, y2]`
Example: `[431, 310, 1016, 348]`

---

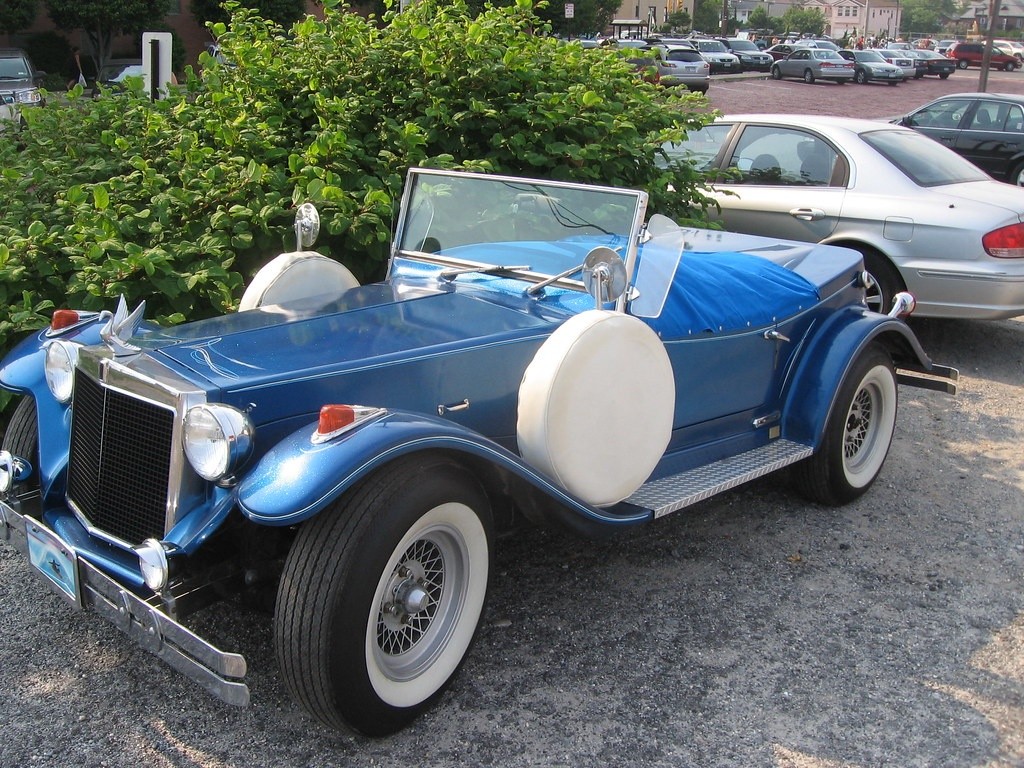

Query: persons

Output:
[750, 33, 903, 51]
[65, 46, 82, 92]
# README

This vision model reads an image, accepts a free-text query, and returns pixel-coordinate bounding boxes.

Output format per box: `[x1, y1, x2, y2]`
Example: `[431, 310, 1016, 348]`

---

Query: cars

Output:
[91, 59, 179, 103]
[635, 94, 1024, 322]
[569, 27, 1023, 93]
[0, 47, 48, 152]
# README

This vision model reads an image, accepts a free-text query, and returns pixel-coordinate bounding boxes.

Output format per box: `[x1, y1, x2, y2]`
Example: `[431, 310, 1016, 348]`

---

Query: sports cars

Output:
[0, 168, 958, 737]
